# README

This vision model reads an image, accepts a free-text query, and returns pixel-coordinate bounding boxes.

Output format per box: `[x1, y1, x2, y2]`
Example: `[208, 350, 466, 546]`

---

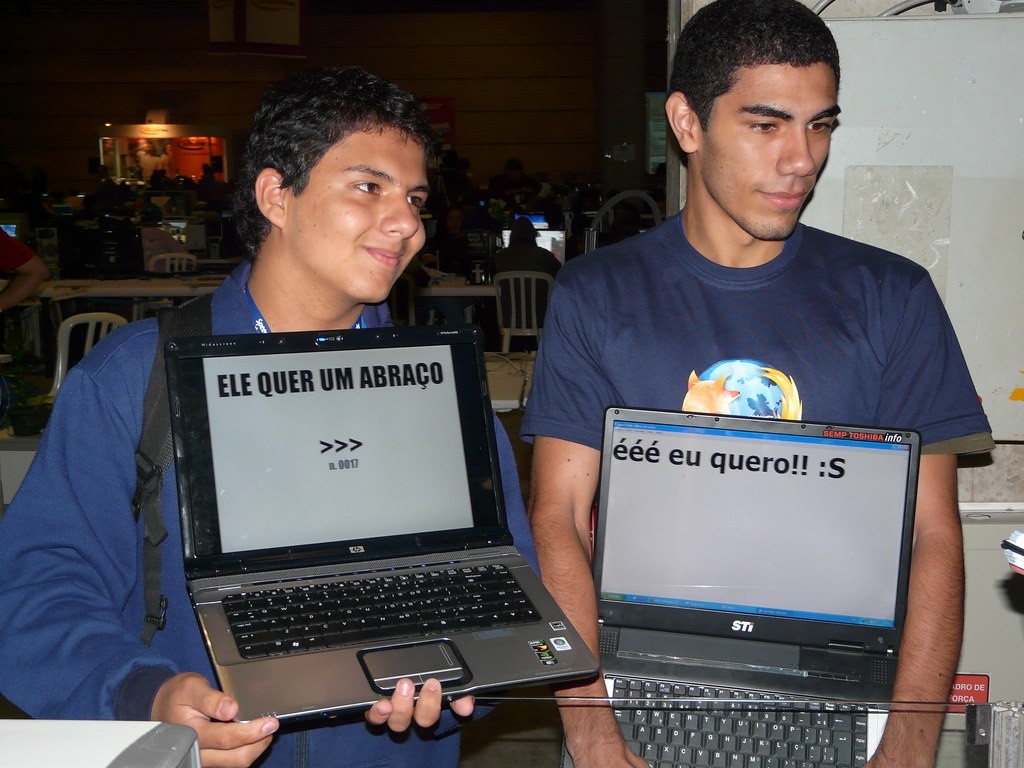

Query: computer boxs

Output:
[35, 218, 66, 281]
[465, 229, 501, 286]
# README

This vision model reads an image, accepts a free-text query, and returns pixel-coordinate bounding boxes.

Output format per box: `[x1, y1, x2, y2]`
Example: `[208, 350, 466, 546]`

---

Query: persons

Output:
[526, 0, 993, 768]
[0, 159, 667, 355]
[0, 55, 543, 768]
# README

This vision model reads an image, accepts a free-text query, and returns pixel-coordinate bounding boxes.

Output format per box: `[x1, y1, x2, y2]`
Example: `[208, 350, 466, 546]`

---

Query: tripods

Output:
[429, 160, 449, 204]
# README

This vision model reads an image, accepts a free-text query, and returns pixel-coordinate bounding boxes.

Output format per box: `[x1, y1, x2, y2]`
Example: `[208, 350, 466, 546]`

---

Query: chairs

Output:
[493, 270, 553, 353]
[132, 253, 196, 321]
[43, 312, 128, 398]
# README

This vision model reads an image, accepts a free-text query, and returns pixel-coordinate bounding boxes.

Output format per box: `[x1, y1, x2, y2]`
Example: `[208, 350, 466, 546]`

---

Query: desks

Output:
[415, 286, 501, 327]
[0, 280, 229, 320]
[198, 255, 245, 264]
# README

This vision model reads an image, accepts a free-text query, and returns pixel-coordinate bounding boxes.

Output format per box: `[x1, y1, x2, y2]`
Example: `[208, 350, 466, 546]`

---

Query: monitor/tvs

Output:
[501, 229, 566, 268]
[513, 211, 550, 230]
[0, 213, 29, 240]
[154, 220, 187, 244]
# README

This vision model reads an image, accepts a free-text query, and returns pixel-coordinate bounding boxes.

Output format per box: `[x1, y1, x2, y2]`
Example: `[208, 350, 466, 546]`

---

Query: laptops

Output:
[560, 404, 921, 768]
[163, 322, 599, 727]
